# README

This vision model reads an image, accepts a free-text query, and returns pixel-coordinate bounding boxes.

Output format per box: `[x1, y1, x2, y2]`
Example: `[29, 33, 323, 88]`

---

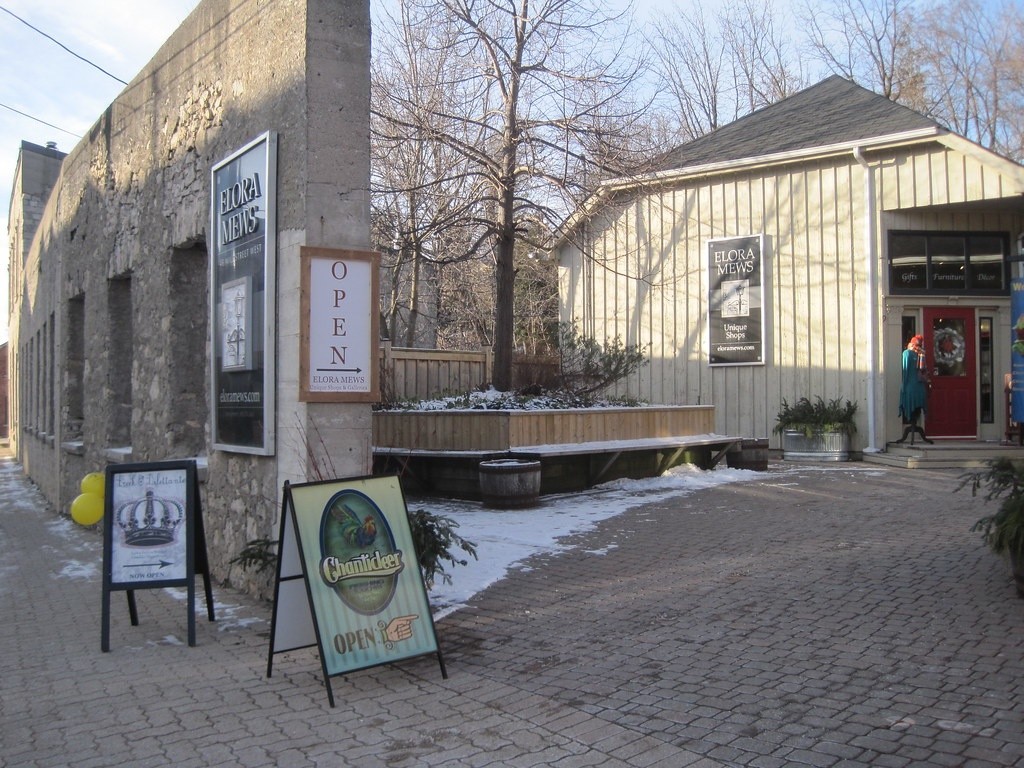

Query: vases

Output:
[478, 458, 542, 507]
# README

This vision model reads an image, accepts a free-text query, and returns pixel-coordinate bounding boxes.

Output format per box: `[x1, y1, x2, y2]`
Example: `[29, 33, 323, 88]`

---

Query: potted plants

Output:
[774, 395, 859, 462]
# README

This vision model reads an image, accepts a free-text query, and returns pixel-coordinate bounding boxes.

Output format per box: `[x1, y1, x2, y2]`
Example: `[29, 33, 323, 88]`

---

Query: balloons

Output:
[71, 473, 105, 524]
